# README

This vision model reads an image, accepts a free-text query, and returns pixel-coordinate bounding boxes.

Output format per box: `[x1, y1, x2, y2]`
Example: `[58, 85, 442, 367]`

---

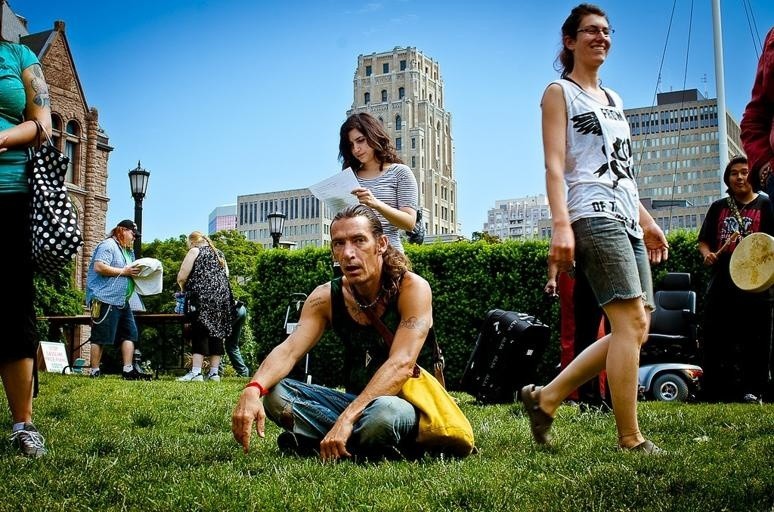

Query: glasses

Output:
[575, 26, 615, 35]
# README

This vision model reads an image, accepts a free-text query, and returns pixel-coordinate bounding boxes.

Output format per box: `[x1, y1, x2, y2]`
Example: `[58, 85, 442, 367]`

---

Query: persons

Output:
[337, 113, 445, 390]
[225, 296, 249, 380]
[739, 25, 774, 198]
[520, 4, 671, 466]
[85, 220, 154, 382]
[229, 203, 445, 466]
[175, 230, 230, 384]
[545, 240, 614, 413]
[1, 3, 55, 461]
[697, 157, 774, 405]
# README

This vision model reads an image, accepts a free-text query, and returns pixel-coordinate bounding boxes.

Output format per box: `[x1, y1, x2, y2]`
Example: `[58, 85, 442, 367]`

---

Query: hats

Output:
[117, 219, 142, 238]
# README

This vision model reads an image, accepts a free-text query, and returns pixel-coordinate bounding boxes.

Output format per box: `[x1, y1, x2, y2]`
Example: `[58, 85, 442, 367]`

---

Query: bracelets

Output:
[241, 379, 269, 401]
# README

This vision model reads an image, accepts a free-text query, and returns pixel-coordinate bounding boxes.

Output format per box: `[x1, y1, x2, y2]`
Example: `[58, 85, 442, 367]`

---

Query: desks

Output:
[34, 313, 189, 380]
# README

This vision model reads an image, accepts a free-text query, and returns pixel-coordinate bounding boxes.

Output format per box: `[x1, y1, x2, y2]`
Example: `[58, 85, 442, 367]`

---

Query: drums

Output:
[727, 233, 774, 291]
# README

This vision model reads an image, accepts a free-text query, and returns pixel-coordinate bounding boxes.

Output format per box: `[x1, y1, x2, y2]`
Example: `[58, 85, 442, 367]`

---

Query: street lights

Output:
[127, 159, 151, 259]
[265, 209, 288, 248]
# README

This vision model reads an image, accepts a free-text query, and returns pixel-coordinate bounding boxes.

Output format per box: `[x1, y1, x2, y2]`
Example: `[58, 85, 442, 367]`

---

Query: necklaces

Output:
[347, 285, 385, 309]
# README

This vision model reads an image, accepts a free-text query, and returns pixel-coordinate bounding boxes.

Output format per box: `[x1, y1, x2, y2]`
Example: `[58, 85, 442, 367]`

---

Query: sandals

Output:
[628, 440, 670, 456]
[522, 384, 556, 446]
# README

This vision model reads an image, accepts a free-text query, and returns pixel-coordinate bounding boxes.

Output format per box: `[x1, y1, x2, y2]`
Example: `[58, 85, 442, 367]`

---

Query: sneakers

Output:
[89, 368, 101, 378]
[122, 365, 154, 380]
[205, 372, 221, 383]
[6, 422, 48, 458]
[176, 370, 204, 382]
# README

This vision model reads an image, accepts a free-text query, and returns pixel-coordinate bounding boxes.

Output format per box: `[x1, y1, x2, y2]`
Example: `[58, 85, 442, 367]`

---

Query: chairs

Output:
[640, 273, 699, 360]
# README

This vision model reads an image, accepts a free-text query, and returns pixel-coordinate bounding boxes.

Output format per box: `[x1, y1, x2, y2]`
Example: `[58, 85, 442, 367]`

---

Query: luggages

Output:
[460, 291, 560, 406]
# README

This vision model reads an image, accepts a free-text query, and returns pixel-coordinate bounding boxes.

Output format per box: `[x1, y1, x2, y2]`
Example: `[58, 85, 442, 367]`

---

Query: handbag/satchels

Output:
[25, 118, 83, 277]
[349, 285, 475, 457]
